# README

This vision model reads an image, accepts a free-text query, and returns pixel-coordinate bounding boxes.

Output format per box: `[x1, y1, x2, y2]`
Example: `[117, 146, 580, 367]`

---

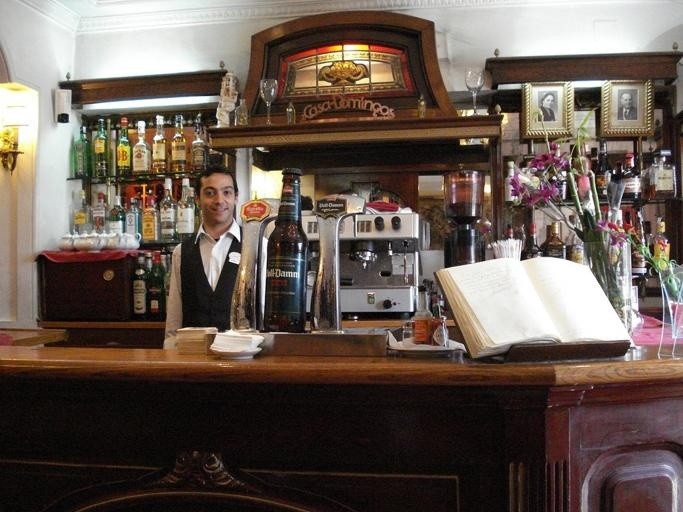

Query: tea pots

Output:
[58, 231, 142, 252]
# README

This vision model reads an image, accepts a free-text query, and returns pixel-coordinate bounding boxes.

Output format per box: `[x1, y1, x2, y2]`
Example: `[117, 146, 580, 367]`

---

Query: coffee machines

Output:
[442, 169, 485, 268]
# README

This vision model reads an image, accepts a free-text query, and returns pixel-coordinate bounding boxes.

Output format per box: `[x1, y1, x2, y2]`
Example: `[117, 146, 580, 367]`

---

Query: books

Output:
[432, 257, 637, 361]
[174, 326, 217, 356]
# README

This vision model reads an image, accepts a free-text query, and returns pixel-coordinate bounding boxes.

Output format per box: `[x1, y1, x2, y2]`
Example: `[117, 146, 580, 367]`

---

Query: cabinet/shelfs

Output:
[39, 114, 225, 328]
[500, 86, 682, 333]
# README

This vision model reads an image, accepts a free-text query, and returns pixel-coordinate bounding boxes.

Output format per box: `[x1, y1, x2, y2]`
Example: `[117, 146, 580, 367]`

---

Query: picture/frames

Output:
[601, 79, 655, 137]
[520, 81, 575, 141]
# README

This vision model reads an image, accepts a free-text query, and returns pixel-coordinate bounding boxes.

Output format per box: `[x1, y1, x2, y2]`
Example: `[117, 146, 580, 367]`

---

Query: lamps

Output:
[0, 81, 41, 173]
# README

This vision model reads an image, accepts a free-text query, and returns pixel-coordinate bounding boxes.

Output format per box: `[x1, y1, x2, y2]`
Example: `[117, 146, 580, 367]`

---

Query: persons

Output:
[538, 92, 555, 122]
[165, 164, 263, 341]
[618, 92, 637, 120]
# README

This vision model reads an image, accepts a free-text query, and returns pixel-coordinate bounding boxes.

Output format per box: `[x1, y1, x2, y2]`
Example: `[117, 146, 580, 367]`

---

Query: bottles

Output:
[264, 168, 309, 333]
[418, 95, 426, 119]
[74, 178, 203, 240]
[234, 99, 250, 127]
[505, 206, 670, 278]
[134, 251, 173, 321]
[286, 102, 296, 125]
[504, 139, 680, 202]
[412, 279, 449, 347]
[73, 116, 225, 177]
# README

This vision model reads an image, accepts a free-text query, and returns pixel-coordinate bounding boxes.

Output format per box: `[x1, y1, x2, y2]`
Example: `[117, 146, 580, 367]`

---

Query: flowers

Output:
[507, 105, 650, 305]
[596, 219, 683, 287]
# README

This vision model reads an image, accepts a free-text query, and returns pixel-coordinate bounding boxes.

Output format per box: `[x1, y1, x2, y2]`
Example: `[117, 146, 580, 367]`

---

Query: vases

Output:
[580, 224, 643, 350]
[654, 268, 683, 362]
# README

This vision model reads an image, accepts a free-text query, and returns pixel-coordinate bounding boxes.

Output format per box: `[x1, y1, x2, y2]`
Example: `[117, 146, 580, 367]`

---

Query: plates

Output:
[214, 347, 262, 360]
[388, 342, 457, 357]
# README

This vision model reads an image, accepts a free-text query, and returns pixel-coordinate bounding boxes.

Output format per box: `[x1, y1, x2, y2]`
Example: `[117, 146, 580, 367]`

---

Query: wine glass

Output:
[260, 79, 278, 127]
[465, 67, 486, 117]
